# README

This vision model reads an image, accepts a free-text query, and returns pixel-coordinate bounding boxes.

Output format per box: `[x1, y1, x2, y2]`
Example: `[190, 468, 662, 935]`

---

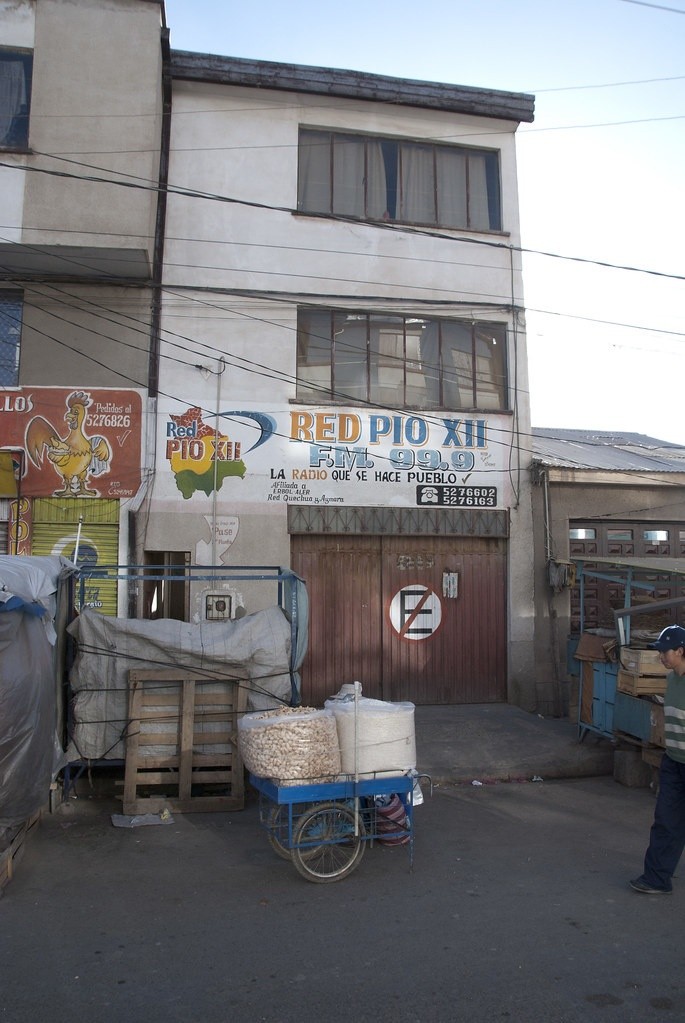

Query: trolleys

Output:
[249, 770, 435, 886]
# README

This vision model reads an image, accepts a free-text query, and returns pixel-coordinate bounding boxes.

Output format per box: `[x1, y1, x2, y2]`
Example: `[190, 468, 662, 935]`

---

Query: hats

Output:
[648, 623, 685, 649]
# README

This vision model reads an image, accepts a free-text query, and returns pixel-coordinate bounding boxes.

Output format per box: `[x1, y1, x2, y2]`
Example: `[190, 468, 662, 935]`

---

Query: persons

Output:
[628, 624, 685, 895]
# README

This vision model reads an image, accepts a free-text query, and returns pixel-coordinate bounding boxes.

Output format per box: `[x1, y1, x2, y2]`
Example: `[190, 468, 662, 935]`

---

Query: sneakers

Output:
[629, 877, 673, 893]
